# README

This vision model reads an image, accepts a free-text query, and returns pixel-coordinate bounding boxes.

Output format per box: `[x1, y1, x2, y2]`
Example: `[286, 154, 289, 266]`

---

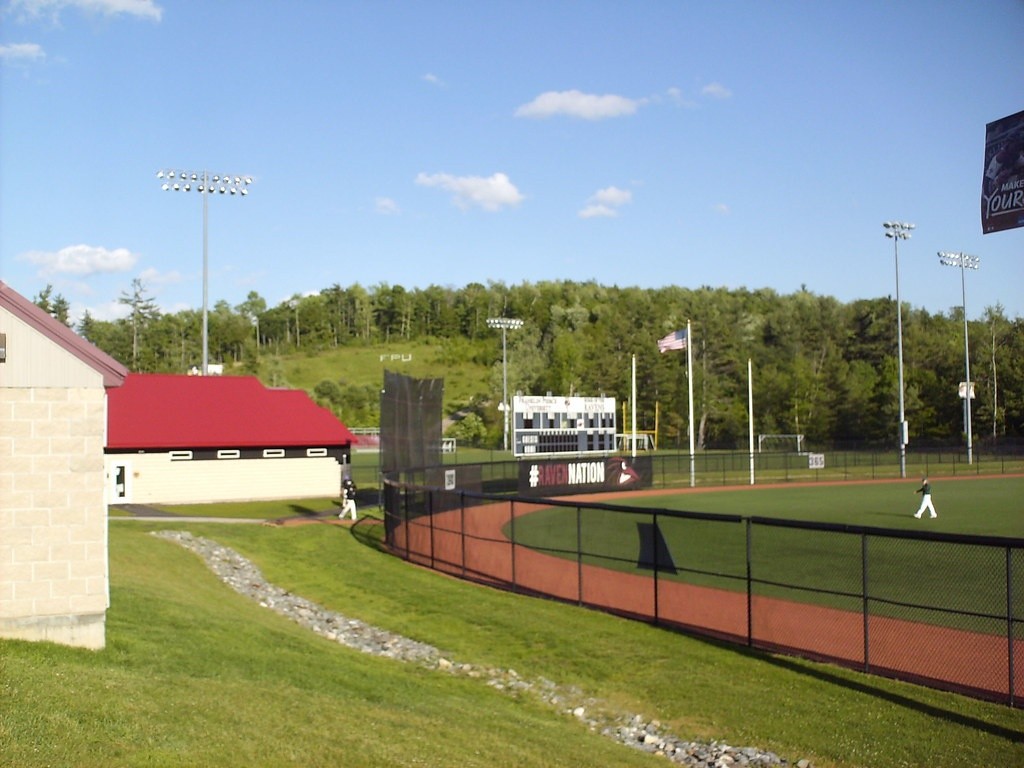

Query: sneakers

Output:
[912, 513, 920, 519]
[929, 514, 937, 518]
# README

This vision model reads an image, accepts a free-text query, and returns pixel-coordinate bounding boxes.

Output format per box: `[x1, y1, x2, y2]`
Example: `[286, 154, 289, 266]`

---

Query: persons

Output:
[913, 477, 937, 519]
[342, 475, 351, 508]
[339, 479, 357, 521]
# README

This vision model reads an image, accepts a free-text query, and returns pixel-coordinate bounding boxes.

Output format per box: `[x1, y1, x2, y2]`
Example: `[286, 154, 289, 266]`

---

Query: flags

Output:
[657, 327, 688, 353]
